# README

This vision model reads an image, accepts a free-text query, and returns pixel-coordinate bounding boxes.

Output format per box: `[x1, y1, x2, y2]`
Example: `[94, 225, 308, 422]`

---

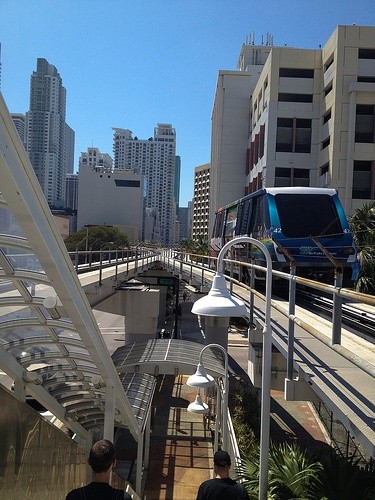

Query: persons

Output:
[196, 451, 249, 500]
[65, 439, 133, 500]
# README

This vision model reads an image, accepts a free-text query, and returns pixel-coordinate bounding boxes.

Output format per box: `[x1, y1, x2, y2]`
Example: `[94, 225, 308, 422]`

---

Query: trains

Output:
[210, 186, 361, 291]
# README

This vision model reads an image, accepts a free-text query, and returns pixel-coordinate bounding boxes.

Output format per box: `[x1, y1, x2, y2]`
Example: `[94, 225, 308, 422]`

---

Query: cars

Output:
[9, 345, 66, 410]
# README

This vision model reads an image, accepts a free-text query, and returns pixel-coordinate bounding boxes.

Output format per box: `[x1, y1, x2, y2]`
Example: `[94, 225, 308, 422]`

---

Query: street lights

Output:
[186, 344, 229, 454]
[187, 381, 221, 480]
[190, 237, 273, 500]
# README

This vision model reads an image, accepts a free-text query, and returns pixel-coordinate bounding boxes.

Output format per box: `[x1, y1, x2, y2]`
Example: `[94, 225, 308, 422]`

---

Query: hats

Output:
[214, 451, 231, 466]
